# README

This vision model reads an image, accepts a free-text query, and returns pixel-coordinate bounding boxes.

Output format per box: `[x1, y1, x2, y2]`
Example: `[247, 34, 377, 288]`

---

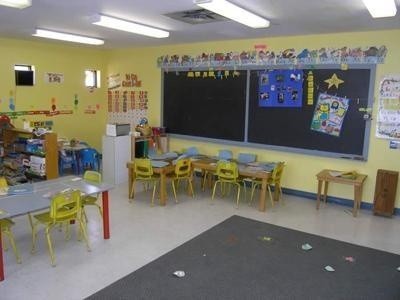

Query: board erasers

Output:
[353, 157, 362, 161]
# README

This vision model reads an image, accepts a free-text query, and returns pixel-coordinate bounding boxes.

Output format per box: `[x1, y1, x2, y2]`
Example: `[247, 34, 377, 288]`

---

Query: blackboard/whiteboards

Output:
[160, 63, 377, 163]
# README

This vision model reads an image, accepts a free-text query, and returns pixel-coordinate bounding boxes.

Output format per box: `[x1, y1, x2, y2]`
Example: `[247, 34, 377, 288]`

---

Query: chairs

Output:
[59, 141, 100, 176]
[126, 147, 286, 212]
[0, 177, 40, 249]
[32, 191, 96, 267]
[0, 218, 21, 264]
[60, 171, 103, 241]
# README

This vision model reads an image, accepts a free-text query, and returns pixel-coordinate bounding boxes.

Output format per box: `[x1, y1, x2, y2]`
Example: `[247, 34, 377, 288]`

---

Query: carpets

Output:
[83, 214, 400, 300]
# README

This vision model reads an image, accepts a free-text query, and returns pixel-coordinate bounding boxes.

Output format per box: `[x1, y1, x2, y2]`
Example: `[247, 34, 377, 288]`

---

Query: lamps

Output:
[0, 0, 31, 9]
[193, 0, 270, 29]
[90, 14, 170, 39]
[31, 28, 104, 46]
[363, 0, 397, 18]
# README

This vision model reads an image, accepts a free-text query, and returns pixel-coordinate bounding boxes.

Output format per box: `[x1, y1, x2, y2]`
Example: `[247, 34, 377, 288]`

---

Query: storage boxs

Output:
[106, 123, 130, 136]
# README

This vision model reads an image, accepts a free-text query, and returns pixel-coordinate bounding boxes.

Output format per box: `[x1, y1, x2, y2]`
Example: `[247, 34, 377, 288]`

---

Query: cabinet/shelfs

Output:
[2, 129, 59, 180]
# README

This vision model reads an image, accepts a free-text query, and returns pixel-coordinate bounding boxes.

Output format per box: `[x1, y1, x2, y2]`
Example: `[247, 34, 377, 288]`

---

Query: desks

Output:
[315, 168, 368, 218]
[0, 175, 116, 282]
